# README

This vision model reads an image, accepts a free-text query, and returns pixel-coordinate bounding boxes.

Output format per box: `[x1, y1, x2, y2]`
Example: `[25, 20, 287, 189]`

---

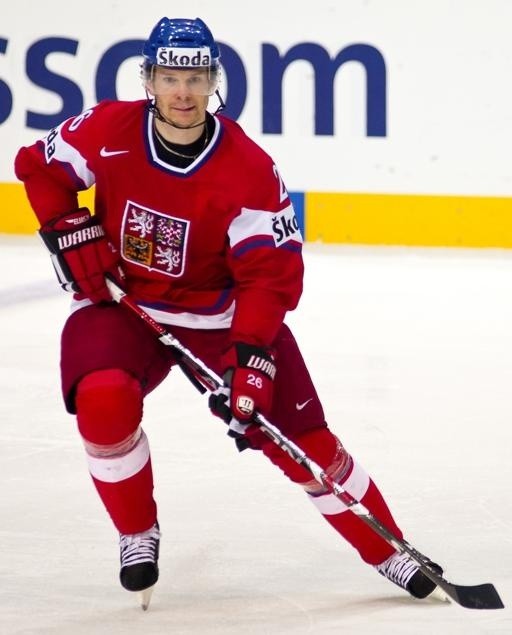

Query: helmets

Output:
[142, 15, 221, 95]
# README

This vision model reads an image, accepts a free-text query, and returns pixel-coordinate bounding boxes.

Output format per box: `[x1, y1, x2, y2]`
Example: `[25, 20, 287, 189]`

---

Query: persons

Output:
[15, 16, 442, 600]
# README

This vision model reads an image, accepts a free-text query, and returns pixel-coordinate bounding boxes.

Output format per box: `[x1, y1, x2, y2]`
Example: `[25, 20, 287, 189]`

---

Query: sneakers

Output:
[373, 546, 442, 600]
[119, 521, 160, 592]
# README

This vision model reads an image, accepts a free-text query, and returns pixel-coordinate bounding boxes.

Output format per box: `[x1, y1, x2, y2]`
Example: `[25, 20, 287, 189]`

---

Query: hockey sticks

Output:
[102, 277, 504, 610]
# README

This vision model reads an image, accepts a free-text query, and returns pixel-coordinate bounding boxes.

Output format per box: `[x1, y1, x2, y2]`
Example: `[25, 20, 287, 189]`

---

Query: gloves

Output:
[212, 342, 276, 438]
[39, 207, 128, 307]
